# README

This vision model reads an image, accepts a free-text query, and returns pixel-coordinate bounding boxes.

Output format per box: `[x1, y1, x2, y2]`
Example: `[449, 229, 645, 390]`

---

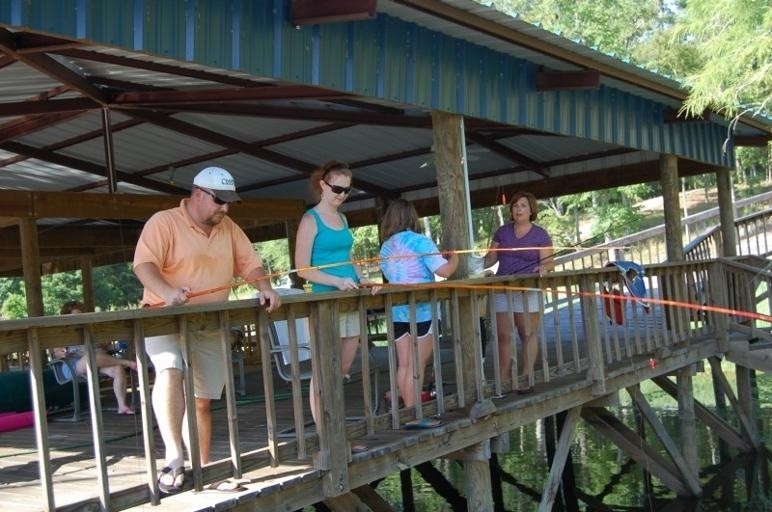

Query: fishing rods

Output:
[353, 283, 772, 323]
[142, 245, 633, 310]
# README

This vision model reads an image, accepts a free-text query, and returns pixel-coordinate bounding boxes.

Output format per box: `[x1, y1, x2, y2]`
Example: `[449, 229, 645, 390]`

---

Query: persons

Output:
[131, 164, 284, 494]
[378, 196, 460, 429]
[480, 189, 555, 394]
[294, 159, 386, 452]
[53, 299, 139, 416]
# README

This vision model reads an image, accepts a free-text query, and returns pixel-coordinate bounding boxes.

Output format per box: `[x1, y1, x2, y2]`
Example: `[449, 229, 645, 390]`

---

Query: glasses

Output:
[325, 181, 351, 194]
[195, 187, 226, 205]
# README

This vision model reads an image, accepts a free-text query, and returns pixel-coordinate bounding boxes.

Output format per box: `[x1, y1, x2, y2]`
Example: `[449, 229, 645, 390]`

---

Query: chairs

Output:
[232, 286, 456, 441]
[45, 341, 139, 423]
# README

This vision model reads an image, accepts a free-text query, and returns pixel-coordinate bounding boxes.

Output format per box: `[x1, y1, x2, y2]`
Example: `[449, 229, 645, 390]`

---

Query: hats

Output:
[193, 167, 243, 204]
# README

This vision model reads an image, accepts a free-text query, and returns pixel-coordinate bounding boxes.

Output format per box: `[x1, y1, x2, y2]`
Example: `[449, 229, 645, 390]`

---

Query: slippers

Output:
[158, 466, 185, 495]
[403, 417, 444, 428]
[117, 408, 135, 416]
